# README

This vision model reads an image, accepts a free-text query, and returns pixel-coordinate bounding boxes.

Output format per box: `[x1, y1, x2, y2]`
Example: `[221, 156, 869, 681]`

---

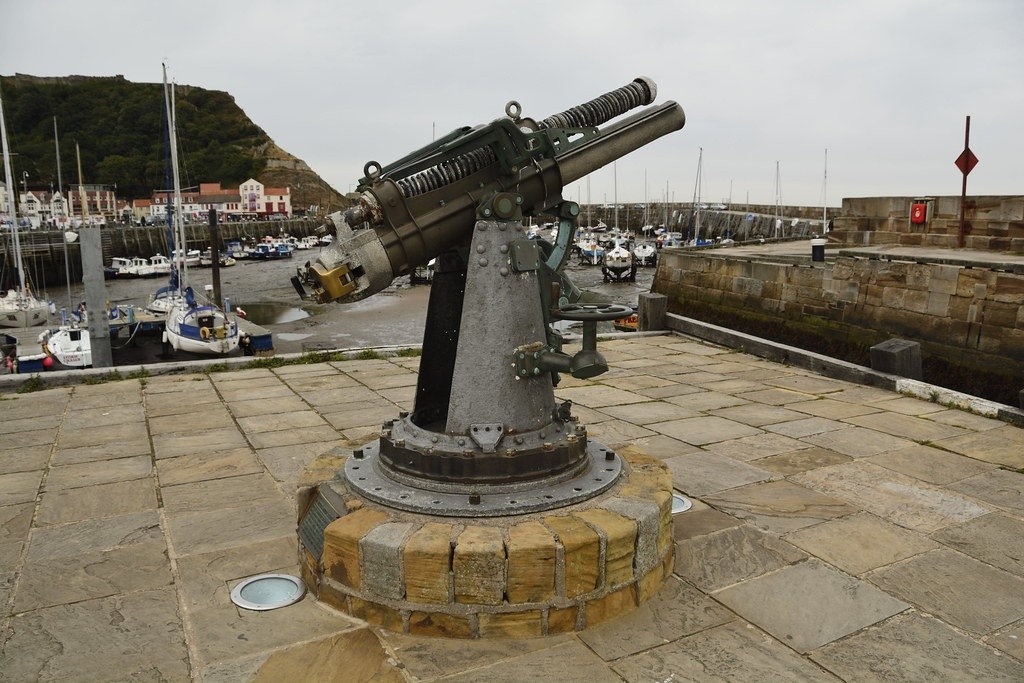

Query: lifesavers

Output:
[199, 326, 209, 339]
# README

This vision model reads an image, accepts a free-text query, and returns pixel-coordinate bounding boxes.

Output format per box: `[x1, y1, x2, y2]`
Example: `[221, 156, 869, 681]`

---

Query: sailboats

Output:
[146, 63, 239, 354]
[46, 116, 92, 367]
[0, 98, 51, 328]
[525, 148, 830, 283]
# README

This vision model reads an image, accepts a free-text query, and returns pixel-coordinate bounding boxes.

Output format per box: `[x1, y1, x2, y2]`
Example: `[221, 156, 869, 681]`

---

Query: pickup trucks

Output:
[146, 215, 165, 226]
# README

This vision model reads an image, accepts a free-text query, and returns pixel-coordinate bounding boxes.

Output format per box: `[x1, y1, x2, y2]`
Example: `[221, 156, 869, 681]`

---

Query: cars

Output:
[274, 213, 286, 221]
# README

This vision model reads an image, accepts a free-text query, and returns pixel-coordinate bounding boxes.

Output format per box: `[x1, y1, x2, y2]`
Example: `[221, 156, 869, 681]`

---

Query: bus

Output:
[230, 213, 258, 222]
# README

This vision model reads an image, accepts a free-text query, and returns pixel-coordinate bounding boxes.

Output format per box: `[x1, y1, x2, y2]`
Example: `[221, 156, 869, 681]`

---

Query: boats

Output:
[103, 234, 334, 280]
